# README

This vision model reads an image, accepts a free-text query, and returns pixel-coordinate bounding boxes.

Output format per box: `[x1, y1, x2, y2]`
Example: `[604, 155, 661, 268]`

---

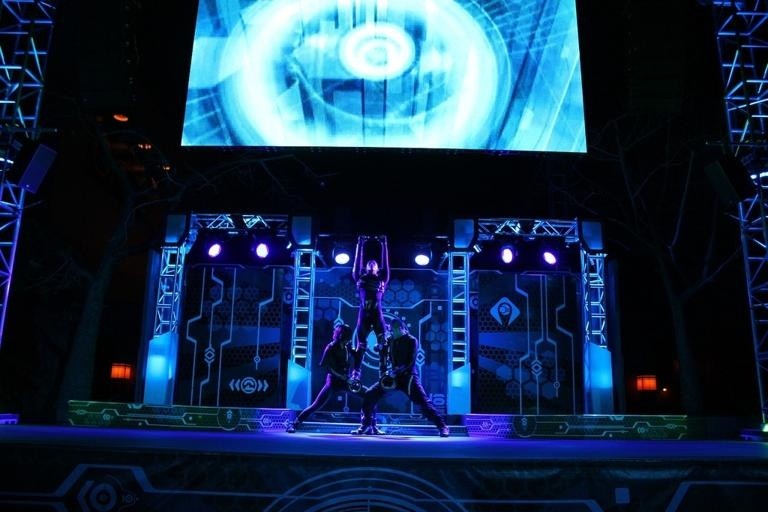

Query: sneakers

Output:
[439, 425, 451, 437]
[349, 425, 386, 435]
[287, 422, 298, 433]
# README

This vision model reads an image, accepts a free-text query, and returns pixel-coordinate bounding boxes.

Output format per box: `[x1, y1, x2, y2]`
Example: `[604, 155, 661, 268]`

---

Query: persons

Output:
[349, 317, 453, 437]
[350, 231, 392, 380]
[286, 320, 382, 435]
